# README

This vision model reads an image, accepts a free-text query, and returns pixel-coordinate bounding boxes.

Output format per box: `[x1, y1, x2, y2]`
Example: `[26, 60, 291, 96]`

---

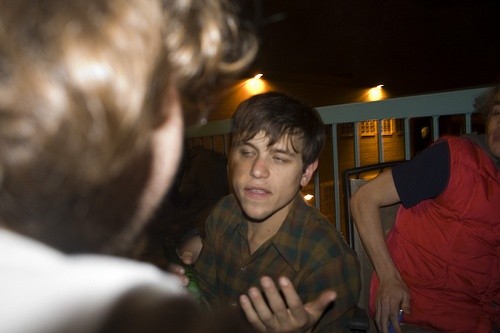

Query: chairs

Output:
[342, 159, 415, 333]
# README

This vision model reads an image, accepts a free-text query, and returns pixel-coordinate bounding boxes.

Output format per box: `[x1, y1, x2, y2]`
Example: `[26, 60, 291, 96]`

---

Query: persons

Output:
[0, 0, 262, 333]
[349, 86, 500, 333]
[116, 91, 361, 333]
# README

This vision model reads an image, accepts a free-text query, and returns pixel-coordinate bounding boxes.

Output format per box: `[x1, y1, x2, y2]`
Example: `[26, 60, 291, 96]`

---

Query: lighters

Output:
[389, 309, 402, 333]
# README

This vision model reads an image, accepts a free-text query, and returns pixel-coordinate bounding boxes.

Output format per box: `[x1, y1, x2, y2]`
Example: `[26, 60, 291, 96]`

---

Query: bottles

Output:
[167, 241, 201, 308]
